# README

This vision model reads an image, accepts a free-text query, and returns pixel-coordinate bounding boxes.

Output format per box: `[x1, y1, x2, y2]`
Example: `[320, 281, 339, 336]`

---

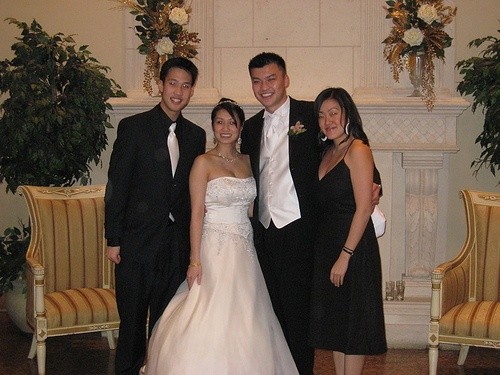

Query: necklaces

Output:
[216, 147, 240, 163]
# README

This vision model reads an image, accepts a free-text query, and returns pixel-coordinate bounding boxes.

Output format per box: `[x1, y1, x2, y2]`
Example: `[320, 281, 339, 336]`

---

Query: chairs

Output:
[428, 188, 500, 375]
[18, 185, 150, 375]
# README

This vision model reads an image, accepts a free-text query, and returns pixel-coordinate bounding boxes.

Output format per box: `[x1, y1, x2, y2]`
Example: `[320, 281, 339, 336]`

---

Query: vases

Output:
[154, 54, 176, 96]
[408, 51, 426, 97]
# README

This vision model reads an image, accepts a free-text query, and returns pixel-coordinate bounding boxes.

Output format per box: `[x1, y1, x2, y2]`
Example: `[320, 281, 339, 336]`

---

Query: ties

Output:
[167, 122, 179, 222]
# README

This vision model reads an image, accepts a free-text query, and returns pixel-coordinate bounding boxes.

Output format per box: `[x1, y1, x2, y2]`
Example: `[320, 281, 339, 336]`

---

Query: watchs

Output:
[378, 184, 381, 188]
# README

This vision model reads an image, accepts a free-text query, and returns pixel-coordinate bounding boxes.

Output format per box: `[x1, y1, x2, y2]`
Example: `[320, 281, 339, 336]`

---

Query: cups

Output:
[396, 281, 405, 301]
[386, 281, 395, 300]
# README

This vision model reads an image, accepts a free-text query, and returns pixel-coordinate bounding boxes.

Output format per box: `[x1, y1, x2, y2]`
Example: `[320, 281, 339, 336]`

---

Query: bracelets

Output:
[344, 246, 353, 251]
[343, 248, 352, 254]
[190, 264, 201, 266]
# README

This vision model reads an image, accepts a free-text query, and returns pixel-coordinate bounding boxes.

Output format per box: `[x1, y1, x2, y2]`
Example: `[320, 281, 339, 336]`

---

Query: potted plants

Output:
[0, 214, 40, 331]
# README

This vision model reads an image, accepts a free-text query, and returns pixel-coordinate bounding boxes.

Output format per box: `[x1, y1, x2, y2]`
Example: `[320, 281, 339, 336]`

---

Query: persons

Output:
[139, 98, 299, 375]
[104, 57, 206, 375]
[240, 53, 383, 375]
[311, 88, 388, 375]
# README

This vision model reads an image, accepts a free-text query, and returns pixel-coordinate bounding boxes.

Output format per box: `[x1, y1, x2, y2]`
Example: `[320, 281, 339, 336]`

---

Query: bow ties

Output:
[262, 111, 279, 120]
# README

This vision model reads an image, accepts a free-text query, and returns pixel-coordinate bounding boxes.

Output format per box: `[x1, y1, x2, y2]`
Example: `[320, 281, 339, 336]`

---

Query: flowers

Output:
[107, 0, 200, 98]
[381, 0, 457, 112]
